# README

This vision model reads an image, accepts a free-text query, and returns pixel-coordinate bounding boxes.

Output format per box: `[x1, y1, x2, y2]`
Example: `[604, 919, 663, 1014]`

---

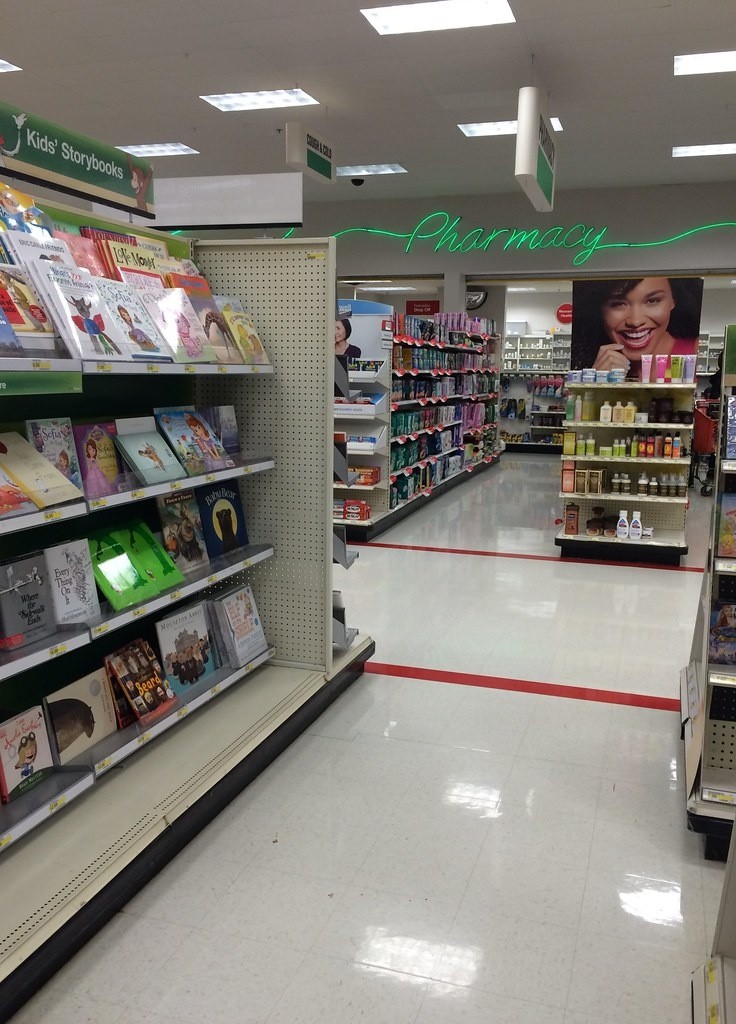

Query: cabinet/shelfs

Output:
[696, 331, 710, 373]
[519, 335, 553, 371]
[0, 102, 378, 983]
[504, 335, 519, 371]
[554, 381, 697, 565]
[333, 298, 506, 543]
[677, 325, 736, 863]
[553, 334, 571, 371]
[707, 334, 725, 372]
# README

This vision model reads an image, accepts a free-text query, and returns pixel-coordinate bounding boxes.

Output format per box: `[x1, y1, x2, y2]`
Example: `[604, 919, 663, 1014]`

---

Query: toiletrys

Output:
[333, 313, 500, 521]
[560, 354, 697, 543]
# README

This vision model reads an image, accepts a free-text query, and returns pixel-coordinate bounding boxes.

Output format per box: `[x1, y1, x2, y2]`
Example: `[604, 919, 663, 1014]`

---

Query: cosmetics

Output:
[563, 430, 689, 459]
[566, 354, 696, 385]
[559, 460, 688, 497]
[566, 391, 696, 425]
[563, 503, 654, 540]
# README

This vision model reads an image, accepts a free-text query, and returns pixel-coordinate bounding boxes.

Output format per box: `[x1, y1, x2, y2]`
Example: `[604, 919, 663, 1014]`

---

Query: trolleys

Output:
[689, 397, 724, 495]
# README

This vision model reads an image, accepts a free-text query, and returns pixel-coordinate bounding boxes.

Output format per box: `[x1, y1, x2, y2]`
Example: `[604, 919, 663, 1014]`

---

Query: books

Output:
[0, 475, 249, 654]
[0, 402, 241, 517]
[0, 181, 274, 366]
[0, 583, 269, 806]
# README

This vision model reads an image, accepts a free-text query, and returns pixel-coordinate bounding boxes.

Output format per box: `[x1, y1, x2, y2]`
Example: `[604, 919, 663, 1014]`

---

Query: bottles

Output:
[611, 473, 687, 497]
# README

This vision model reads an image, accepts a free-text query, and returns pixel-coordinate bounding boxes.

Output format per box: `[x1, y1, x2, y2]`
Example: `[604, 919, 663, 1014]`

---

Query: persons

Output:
[589, 276, 697, 383]
[334, 319, 361, 360]
[704, 349, 723, 480]
